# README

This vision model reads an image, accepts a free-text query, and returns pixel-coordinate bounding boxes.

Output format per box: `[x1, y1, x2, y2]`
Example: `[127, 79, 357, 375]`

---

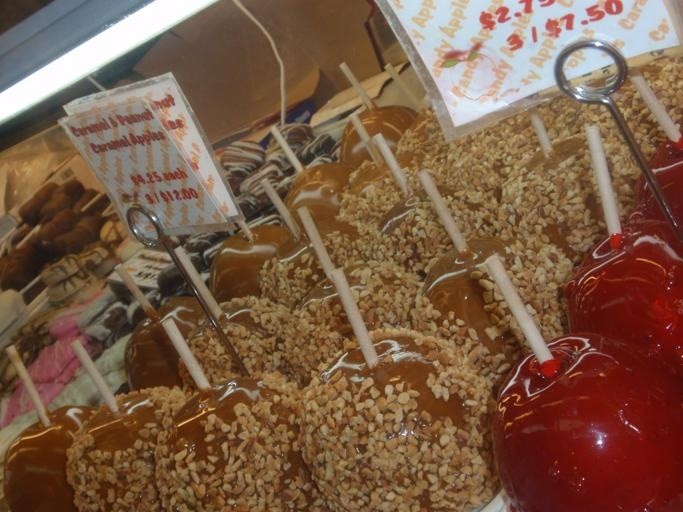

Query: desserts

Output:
[0, 57, 683, 512]
